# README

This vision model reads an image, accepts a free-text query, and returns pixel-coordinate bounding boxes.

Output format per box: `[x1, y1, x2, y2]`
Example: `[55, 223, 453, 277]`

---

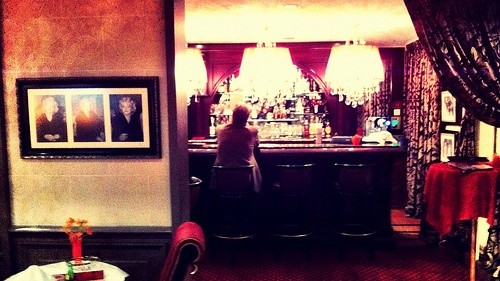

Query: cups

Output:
[351, 137, 361, 145]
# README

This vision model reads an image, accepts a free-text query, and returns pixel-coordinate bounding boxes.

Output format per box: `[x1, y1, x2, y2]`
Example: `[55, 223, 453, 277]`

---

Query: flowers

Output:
[63, 217, 92, 242]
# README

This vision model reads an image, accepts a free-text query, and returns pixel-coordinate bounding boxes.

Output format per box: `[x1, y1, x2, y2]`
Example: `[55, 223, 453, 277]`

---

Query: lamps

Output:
[184, 48, 207, 106]
[235, 24, 300, 109]
[324, 23, 385, 108]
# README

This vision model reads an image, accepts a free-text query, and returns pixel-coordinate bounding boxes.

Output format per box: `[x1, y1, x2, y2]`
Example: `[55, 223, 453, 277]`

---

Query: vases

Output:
[70, 238, 82, 259]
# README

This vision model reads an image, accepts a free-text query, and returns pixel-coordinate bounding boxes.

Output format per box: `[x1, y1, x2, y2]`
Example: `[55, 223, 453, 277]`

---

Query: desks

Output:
[3, 260, 129, 281]
[422, 163, 500, 281]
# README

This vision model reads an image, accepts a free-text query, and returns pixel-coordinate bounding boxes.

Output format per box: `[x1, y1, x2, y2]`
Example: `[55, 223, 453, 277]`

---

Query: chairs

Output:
[209, 165, 262, 240]
[331, 163, 387, 240]
[154, 221, 206, 281]
[271, 163, 319, 239]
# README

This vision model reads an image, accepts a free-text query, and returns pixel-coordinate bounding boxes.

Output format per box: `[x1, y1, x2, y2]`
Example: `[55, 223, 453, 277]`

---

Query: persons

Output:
[112, 97, 144, 141]
[36, 96, 66, 141]
[210, 106, 262, 193]
[75, 97, 105, 142]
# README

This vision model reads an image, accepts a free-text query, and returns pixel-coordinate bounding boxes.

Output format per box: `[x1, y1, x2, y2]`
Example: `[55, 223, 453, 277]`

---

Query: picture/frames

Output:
[16, 76, 162, 159]
